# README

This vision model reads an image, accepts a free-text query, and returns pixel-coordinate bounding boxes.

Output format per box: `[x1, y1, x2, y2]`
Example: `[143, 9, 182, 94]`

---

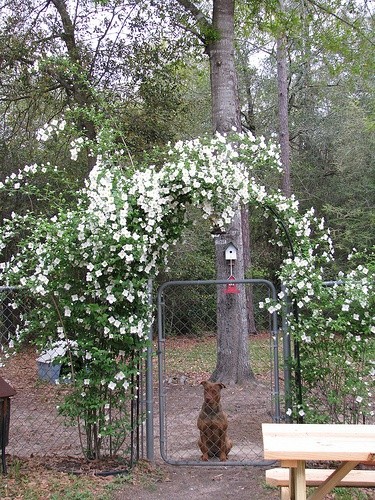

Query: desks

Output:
[262, 423, 375, 500]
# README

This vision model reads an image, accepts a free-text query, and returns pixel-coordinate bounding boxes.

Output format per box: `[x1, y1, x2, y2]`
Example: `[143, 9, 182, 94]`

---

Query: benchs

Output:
[265, 467, 375, 500]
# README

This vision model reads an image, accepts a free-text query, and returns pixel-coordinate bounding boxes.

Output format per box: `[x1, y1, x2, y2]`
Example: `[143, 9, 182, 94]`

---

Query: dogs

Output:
[196, 380, 233, 462]
